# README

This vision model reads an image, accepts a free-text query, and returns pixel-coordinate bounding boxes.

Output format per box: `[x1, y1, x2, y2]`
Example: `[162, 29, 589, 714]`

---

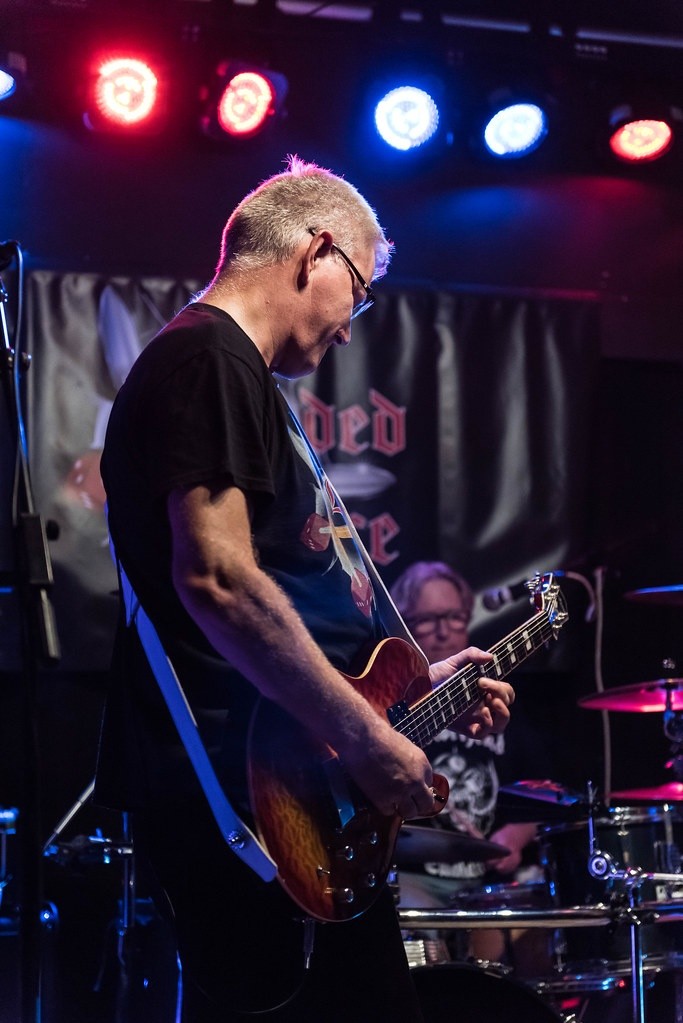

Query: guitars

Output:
[222, 573, 572, 925]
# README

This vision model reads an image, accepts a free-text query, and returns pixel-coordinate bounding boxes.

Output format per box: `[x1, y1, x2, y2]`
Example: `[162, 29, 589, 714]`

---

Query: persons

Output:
[98, 158, 516, 1023]
[389, 561, 564, 969]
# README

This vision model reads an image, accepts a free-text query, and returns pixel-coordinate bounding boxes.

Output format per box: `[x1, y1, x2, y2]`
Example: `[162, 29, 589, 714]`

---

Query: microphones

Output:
[0, 239, 19, 273]
[479, 579, 530, 612]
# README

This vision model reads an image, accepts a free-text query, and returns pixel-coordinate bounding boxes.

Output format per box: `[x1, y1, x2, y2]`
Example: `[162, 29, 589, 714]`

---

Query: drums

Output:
[410, 958, 580, 1023]
[447, 878, 559, 981]
[522, 952, 683, 1023]
[535, 809, 683, 968]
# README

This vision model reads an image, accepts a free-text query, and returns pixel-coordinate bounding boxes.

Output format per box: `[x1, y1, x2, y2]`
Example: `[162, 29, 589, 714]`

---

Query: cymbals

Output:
[606, 781, 683, 802]
[625, 583, 683, 602]
[498, 775, 580, 808]
[394, 821, 512, 864]
[576, 674, 683, 714]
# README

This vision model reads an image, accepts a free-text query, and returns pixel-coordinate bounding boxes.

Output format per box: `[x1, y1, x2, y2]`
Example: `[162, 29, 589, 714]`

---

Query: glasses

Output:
[310, 226, 376, 322]
[410, 609, 471, 637]
